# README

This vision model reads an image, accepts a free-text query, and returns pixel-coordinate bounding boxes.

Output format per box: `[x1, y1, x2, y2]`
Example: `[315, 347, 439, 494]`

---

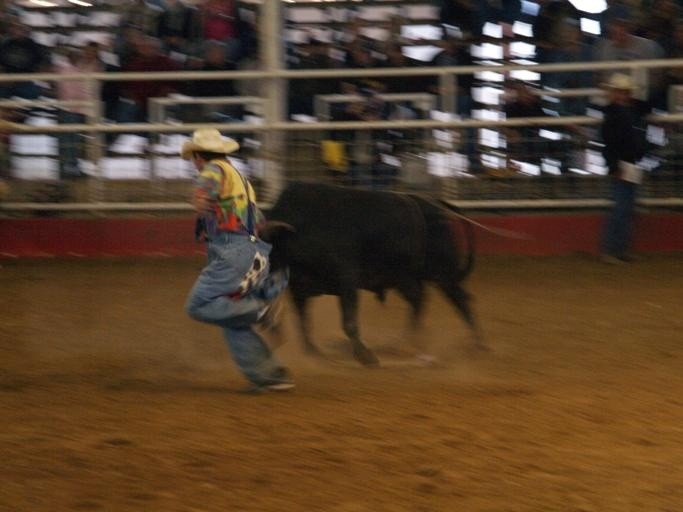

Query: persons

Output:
[597, 72, 647, 265]
[1, 1, 683, 179]
[178, 129, 299, 395]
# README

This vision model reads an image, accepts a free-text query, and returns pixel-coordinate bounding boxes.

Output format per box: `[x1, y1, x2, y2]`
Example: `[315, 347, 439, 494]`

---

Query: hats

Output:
[179, 129, 240, 159]
[599, 73, 640, 90]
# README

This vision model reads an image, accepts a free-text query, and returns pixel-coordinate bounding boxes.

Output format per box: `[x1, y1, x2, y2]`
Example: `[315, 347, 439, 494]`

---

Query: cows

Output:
[255, 176, 479, 369]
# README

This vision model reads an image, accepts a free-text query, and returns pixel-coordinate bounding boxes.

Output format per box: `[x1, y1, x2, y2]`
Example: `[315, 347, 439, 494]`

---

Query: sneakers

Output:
[253, 378, 295, 392]
[259, 303, 284, 331]
